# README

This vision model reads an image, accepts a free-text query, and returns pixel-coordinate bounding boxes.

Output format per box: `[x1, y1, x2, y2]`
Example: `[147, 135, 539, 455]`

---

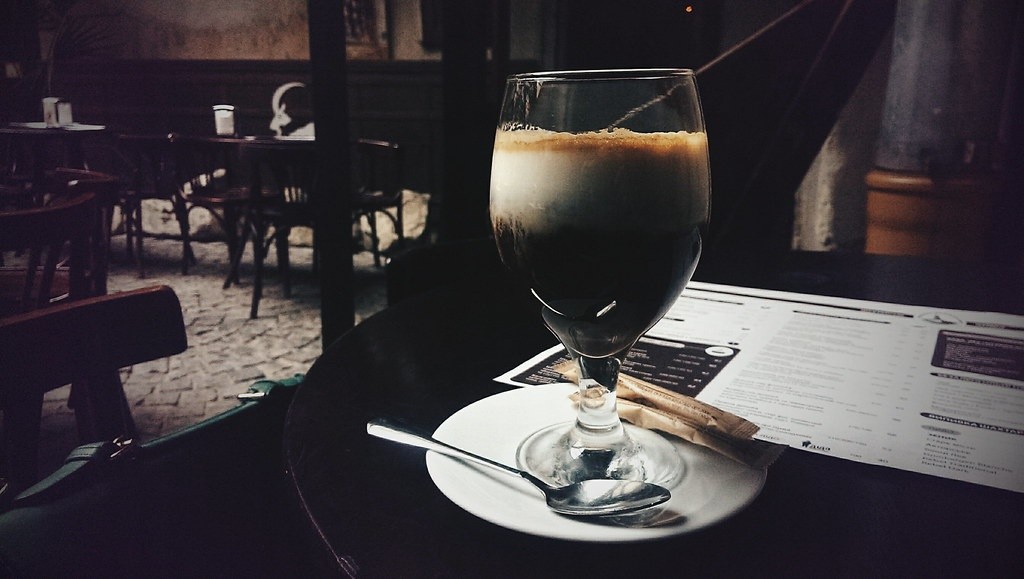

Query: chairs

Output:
[0, 131, 402, 497]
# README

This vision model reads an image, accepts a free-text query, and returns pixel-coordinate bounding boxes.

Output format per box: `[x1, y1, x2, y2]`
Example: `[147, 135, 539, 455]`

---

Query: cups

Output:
[57, 102, 73, 127]
[42, 96, 59, 126]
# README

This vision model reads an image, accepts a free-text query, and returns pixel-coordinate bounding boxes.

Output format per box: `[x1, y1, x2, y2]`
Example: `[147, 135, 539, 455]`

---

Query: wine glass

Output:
[489, 64, 711, 498]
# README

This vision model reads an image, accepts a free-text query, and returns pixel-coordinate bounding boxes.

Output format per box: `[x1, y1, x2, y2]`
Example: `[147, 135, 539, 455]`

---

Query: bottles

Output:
[213, 105, 234, 137]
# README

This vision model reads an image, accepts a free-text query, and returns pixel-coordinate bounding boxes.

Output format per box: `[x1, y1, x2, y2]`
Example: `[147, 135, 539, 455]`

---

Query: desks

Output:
[280, 279, 1023, 579]
[205, 137, 315, 274]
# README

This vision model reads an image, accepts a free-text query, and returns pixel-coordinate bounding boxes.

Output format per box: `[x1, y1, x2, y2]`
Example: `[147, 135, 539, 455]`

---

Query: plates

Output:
[425, 383, 770, 542]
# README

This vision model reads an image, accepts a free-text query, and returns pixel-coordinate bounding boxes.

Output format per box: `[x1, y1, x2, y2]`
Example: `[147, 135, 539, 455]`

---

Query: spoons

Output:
[363, 420, 674, 521]
